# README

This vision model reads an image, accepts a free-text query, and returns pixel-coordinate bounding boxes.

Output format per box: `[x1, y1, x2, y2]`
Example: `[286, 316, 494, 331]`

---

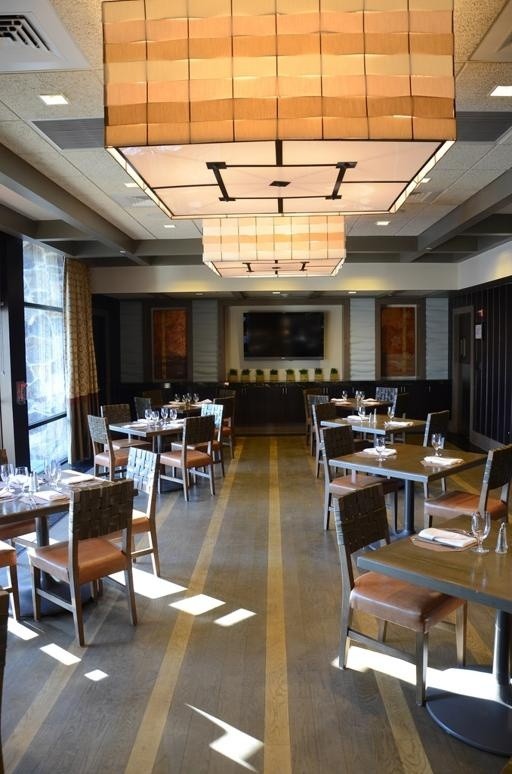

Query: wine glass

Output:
[387, 407, 395, 421]
[342, 391, 347, 400]
[145, 408, 177, 427]
[174, 393, 200, 405]
[374, 437, 386, 462]
[358, 406, 366, 422]
[0, 456, 62, 493]
[355, 391, 365, 402]
[470, 511, 491, 554]
[431, 433, 442, 457]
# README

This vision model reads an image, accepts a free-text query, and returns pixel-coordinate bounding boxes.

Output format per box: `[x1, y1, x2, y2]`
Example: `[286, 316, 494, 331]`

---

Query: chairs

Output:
[0, 447, 159, 646]
[86, 397, 236, 501]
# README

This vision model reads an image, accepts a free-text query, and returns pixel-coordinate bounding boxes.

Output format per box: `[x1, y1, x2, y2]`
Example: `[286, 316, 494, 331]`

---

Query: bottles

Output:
[28, 471, 39, 491]
[369, 413, 377, 423]
[495, 522, 509, 554]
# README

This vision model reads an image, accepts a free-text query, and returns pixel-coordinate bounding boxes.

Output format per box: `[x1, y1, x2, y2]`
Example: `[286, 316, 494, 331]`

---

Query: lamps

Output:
[202, 215, 346, 279]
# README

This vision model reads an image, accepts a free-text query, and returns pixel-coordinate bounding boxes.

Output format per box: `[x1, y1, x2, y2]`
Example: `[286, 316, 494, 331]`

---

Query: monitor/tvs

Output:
[241, 311, 325, 361]
